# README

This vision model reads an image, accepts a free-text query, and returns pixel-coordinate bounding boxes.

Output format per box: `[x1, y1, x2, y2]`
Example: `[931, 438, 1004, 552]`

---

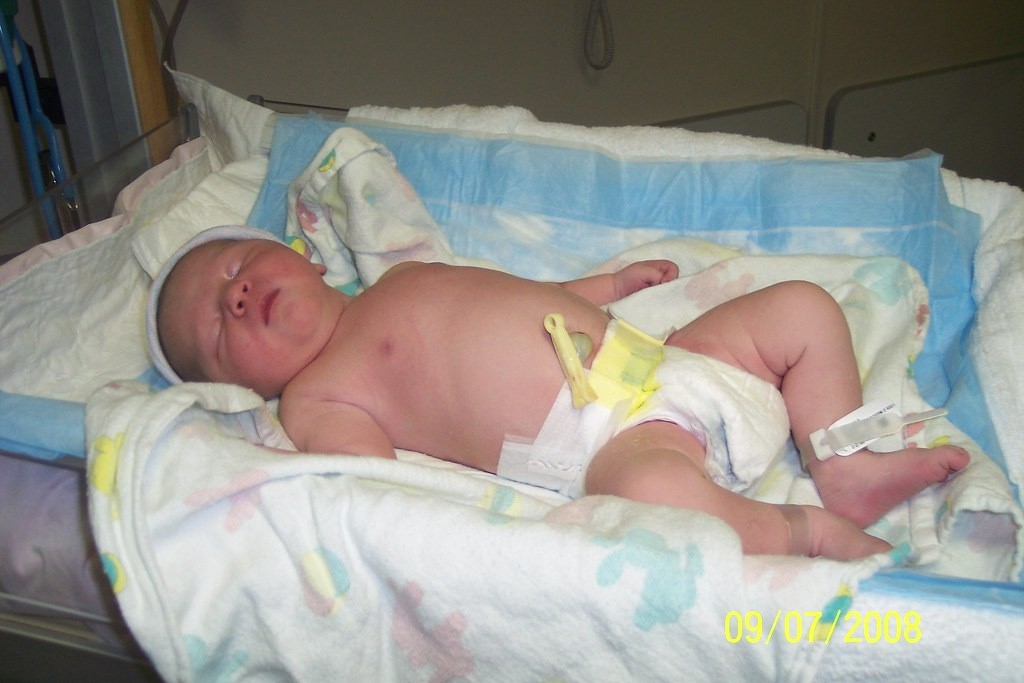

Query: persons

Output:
[146, 223, 969, 563]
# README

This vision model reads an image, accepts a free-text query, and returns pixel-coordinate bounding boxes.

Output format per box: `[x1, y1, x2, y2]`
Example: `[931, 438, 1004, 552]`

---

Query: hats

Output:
[145, 225, 309, 386]
